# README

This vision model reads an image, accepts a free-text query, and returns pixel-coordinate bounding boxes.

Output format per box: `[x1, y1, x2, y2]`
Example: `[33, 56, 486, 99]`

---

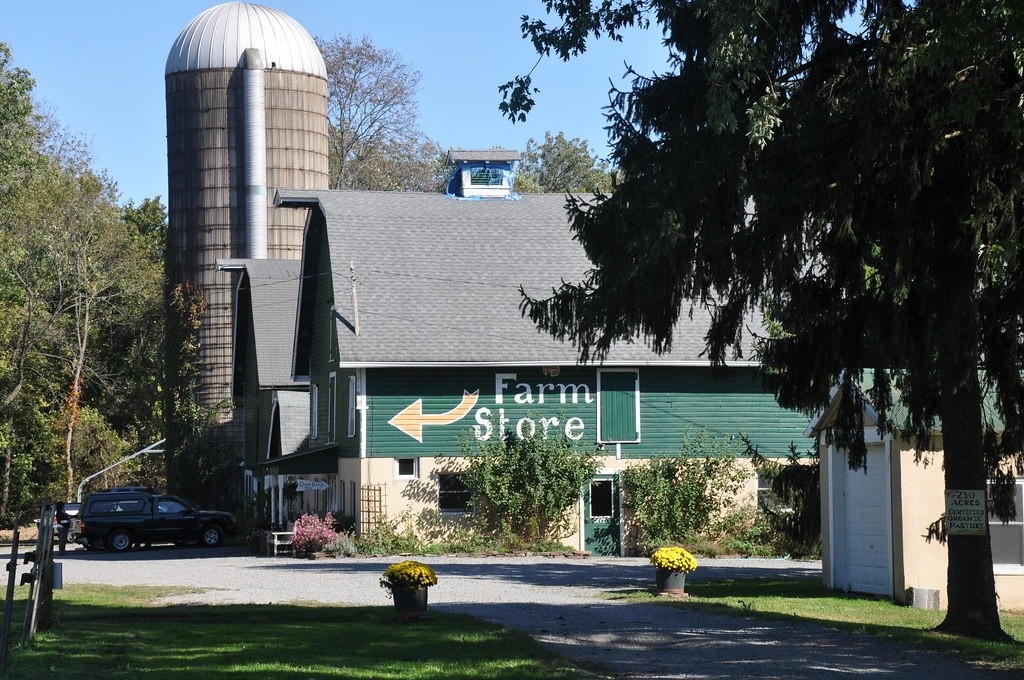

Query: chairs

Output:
[267, 520, 298, 557]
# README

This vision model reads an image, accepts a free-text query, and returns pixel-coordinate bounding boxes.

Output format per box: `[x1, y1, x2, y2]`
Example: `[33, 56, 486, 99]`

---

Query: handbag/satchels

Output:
[59, 523, 70, 528]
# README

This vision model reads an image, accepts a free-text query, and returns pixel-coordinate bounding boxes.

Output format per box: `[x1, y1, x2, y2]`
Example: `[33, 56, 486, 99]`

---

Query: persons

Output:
[56, 501, 79, 551]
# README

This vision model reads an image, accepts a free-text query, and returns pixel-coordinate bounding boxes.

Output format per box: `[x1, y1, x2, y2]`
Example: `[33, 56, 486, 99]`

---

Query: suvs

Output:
[34, 500, 82, 544]
[78, 487, 239, 553]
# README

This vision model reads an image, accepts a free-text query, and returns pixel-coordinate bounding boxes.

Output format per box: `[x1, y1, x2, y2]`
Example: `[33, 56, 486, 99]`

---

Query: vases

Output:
[655, 569, 685, 593]
[390, 586, 428, 611]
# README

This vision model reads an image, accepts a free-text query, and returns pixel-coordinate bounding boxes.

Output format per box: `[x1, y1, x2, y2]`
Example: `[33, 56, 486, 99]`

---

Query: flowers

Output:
[650, 545, 697, 573]
[379, 561, 437, 599]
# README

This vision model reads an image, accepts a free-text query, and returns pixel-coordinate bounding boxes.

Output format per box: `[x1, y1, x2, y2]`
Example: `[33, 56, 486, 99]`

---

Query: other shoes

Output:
[60, 551, 68, 556]
